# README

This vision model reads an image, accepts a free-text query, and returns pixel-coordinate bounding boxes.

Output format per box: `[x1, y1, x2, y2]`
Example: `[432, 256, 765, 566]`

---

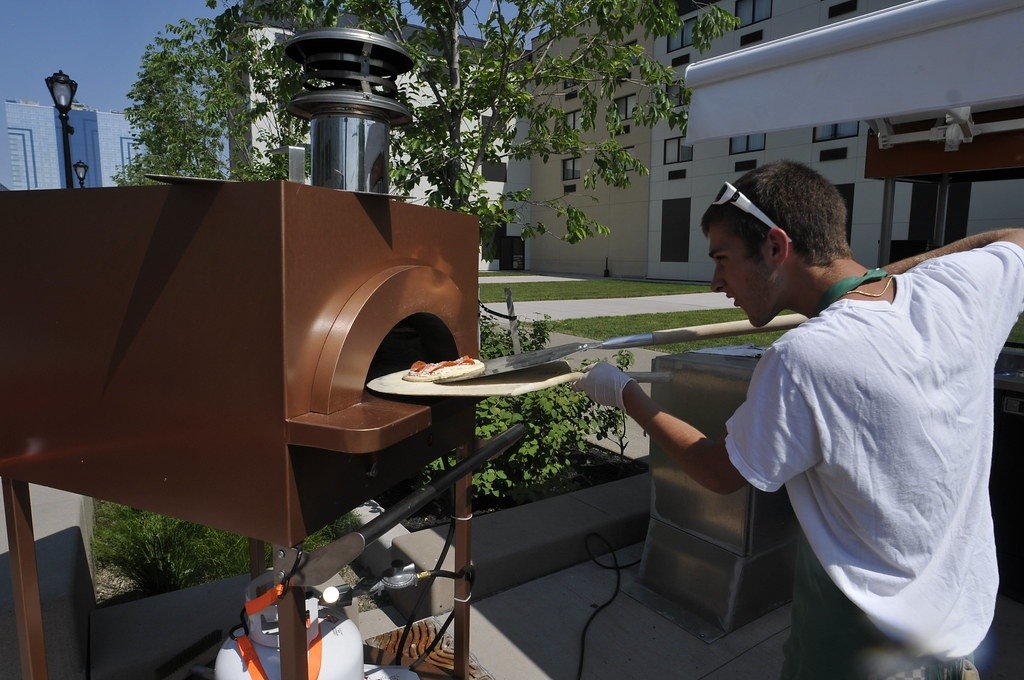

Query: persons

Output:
[567, 154, 1023, 680]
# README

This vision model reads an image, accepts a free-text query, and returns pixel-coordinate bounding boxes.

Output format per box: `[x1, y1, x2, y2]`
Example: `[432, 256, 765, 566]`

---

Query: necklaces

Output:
[842, 275, 896, 299]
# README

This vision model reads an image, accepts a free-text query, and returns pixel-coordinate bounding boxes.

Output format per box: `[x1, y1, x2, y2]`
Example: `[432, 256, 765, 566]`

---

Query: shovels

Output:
[432, 313, 815, 387]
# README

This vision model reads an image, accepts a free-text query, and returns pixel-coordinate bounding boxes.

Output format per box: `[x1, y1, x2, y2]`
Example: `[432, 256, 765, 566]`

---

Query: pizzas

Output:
[403, 354, 487, 382]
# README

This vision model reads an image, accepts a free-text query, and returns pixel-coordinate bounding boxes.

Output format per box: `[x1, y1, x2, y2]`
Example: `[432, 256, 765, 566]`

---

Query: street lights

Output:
[72, 160, 89, 189]
[43, 69, 80, 189]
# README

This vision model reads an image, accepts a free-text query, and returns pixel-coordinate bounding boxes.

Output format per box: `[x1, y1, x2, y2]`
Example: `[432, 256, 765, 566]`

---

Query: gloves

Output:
[572, 361, 637, 415]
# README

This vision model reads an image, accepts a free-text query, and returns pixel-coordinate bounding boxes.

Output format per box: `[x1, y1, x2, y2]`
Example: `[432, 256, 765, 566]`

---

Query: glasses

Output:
[711, 182, 792, 243]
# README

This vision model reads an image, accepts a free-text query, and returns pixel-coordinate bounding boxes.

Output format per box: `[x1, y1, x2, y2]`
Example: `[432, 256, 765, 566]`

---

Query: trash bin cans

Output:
[615, 344, 803, 647]
